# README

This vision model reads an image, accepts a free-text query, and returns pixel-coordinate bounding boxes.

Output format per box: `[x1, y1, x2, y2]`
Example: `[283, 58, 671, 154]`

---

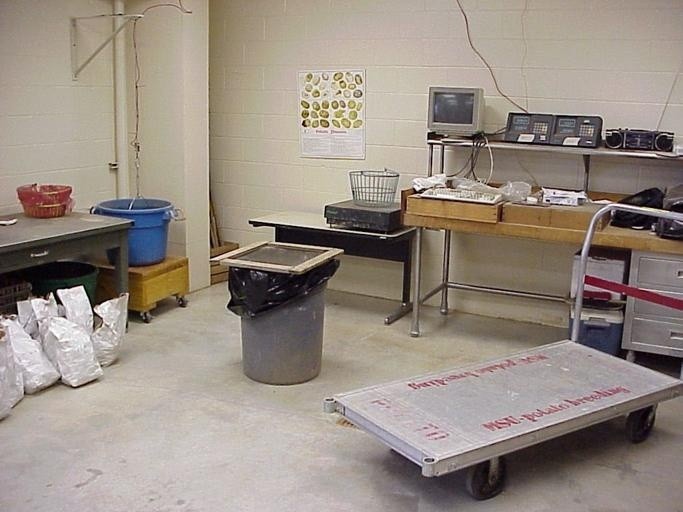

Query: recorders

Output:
[604, 129, 674, 152]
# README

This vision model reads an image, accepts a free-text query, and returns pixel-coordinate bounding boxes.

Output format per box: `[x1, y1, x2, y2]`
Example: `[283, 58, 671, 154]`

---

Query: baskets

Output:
[16, 183, 72, 219]
[349, 170, 399, 208]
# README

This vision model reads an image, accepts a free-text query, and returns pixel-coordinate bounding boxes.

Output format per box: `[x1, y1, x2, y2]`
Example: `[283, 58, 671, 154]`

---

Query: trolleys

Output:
[321, 202, 682, 502]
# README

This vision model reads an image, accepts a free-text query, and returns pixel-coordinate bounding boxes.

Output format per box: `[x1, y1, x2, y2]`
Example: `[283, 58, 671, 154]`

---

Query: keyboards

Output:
[419, 186, 504, 205]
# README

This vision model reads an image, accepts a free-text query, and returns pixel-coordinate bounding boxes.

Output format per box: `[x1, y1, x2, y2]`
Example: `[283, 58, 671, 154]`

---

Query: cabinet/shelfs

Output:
[620, 249, 682, 363]
[424, 138, 683, 207]
[82, 252, 191, 319]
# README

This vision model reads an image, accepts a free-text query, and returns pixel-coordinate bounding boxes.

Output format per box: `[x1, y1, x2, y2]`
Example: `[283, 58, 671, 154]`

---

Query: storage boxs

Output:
[567, 306, 622, 354]
[569, 247, 629, 300]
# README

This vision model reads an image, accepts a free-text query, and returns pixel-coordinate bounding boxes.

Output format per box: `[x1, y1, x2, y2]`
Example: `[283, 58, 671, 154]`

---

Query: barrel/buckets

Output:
[25, 262, 100, 308]
[89, 197, 186, 267]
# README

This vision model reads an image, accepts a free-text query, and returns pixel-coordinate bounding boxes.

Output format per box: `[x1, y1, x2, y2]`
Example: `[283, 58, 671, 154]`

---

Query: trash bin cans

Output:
[228, 245, 328, 385]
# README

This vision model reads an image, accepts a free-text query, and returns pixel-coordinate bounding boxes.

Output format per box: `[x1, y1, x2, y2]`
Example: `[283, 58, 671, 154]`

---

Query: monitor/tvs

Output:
[427, 87, 484, 143]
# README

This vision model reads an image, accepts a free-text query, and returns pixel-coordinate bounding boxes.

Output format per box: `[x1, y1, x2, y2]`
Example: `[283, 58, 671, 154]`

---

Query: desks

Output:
[399, 209, 682, 378]
[0, 211, 134, 331]
[248, 209, 415, 323]
